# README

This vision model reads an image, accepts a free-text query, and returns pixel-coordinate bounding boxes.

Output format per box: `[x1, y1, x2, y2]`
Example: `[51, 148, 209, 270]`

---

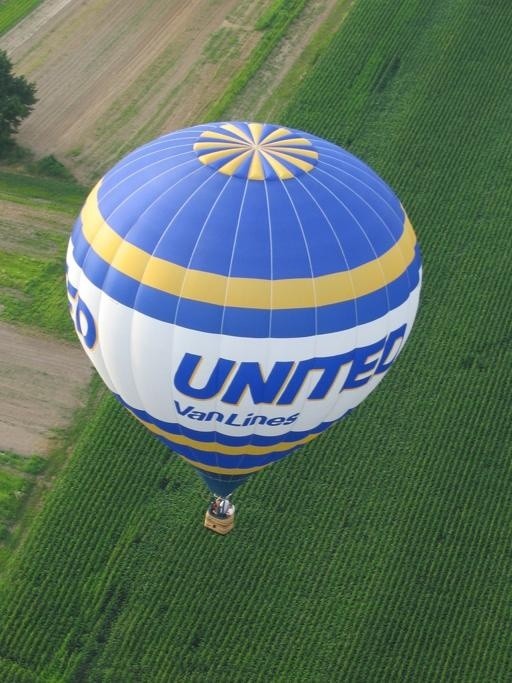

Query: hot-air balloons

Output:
[66, 120, 424, 537]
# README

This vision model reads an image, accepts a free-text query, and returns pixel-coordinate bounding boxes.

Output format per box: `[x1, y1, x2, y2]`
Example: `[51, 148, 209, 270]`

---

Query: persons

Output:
[211, 498, 235, 516]
